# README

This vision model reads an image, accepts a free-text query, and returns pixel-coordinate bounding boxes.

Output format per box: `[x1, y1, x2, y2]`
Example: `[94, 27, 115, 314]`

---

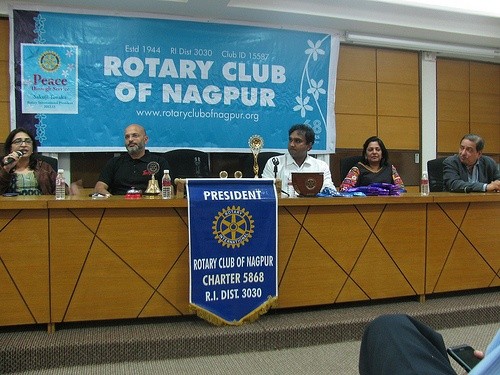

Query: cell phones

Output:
[447, 344, 481, 373]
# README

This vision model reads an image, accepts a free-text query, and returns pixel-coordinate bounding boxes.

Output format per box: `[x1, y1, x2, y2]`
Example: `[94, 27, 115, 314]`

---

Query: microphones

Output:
[3, 150, 23, 165]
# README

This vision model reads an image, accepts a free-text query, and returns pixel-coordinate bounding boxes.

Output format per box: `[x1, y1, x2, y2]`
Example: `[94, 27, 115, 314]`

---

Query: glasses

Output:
[11, 138, 33, 145]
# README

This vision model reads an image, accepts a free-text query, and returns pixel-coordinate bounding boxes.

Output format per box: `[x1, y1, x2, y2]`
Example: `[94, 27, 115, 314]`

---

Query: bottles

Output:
[162, 170, 171, 199]
[56, 169, 65, 200]
[421, 171, 429, 196]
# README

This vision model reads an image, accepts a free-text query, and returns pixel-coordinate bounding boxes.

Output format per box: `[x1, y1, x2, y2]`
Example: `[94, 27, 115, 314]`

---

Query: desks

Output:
[0, 192, 500, 335]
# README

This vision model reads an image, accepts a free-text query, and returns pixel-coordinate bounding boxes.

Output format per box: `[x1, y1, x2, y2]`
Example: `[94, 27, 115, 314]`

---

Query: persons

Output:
[339, 136, 404, 191]
[359, 314, 500, 375]
[442, 134, 500, 192]
[261, 124, 337, 195]
[95, 124, 176, 195]
[0, 127, 79, 195]
